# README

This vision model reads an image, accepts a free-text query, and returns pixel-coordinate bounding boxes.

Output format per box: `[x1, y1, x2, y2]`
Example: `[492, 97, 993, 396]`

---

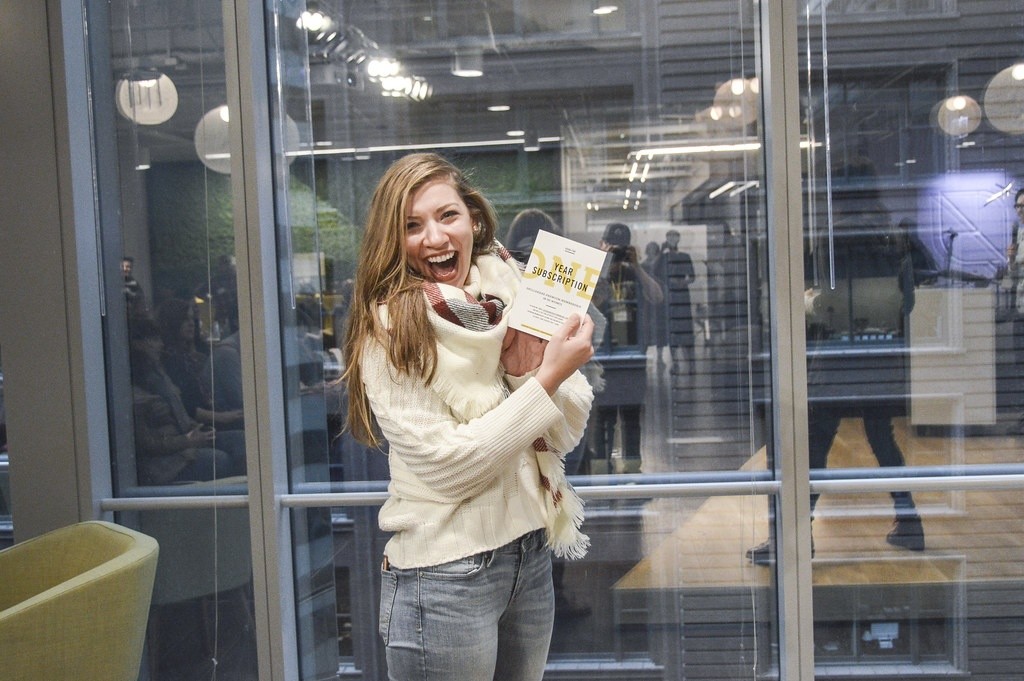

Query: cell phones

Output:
[612, 245, 632, 263]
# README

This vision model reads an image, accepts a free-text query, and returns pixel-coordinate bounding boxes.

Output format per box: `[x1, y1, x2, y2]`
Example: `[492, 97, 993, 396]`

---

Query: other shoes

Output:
[887, 516, 924, 549]
[748, 527, 815, 564]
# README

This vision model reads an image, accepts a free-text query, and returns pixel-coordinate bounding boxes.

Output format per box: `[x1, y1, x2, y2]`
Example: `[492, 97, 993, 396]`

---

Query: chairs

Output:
[0, 520, 157, 681]
[135, 475, 255, 645]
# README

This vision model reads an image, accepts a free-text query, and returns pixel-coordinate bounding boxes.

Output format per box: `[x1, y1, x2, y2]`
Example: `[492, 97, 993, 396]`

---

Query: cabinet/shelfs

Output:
[905, 287, 995, 433]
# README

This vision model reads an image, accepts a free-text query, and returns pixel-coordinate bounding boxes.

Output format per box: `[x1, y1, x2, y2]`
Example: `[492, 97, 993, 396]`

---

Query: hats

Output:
[603, 225, 632, 248]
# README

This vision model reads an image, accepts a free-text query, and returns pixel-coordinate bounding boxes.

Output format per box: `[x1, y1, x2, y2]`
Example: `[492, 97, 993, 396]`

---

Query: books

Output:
[504, 229, 608, 343]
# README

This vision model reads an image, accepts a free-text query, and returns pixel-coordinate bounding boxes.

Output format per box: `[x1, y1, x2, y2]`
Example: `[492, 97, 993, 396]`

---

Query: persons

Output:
[638, 230, 698, 381]
[736, 161, 1023, 566]
[586, 220, 664, 469]
[116, 245, 356, 652]
[338, 154, 594, 680]
[502, 208, 567, 261]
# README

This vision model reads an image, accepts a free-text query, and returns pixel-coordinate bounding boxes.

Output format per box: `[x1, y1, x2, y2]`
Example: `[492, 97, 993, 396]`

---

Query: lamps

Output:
[984, 64, 1024, 135]
[195, 96, 298, 177]
[292, 3, 432, 101]
[714, 76, 760, 126]
[526, 130, 539, 153]
[940, 95, 981, 136]
[118, 70, 177, 126]
[450, 53, 482, 78]
[632, 135, 818, 157]
